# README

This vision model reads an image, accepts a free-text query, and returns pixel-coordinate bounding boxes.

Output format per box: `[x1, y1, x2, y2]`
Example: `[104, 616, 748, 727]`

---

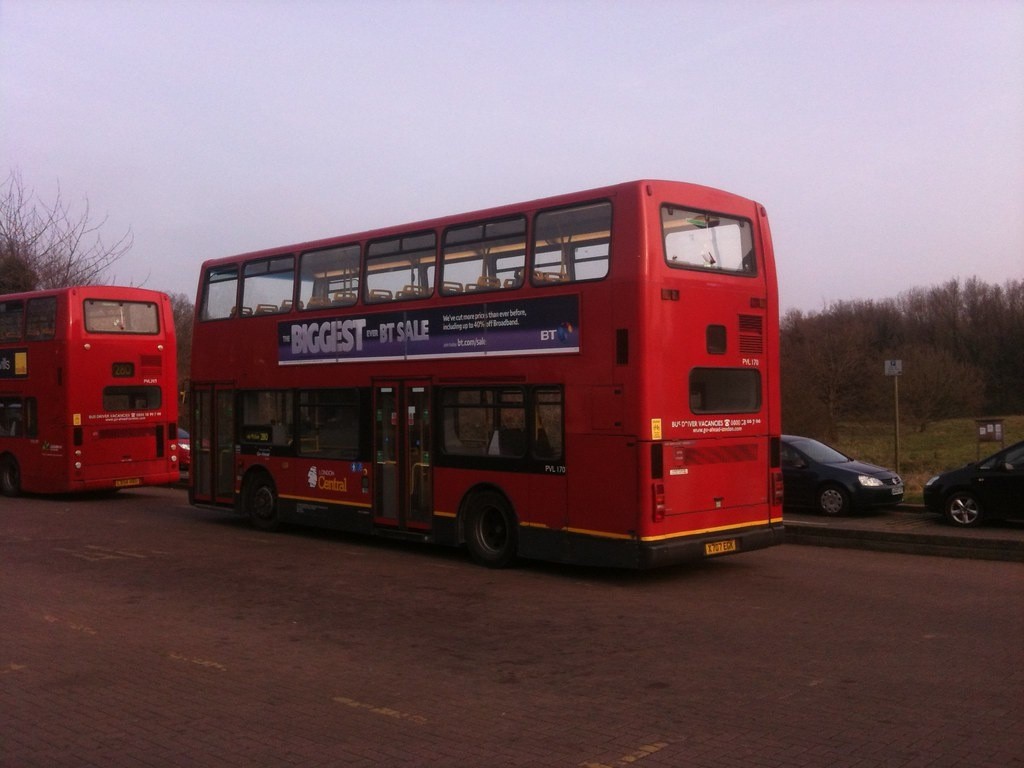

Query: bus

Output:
[179, 178, 788, 571]
[0, 284, 182, 498]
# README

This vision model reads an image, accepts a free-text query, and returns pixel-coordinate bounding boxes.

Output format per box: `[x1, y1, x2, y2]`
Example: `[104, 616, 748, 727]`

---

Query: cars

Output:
[780, 433, 904, 519]
[177, 426, 211, 470]
[922, 439, 1024, 528]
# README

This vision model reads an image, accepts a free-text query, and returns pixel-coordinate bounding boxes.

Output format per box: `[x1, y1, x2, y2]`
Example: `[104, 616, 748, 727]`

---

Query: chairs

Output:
[2, 326, 56, 337]
[230, 269, 571, 319]
